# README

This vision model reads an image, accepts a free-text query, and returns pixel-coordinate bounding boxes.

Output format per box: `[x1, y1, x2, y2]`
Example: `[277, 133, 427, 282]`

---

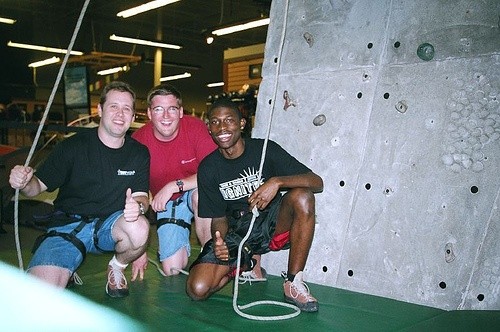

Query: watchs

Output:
[176, 179, 184, 192]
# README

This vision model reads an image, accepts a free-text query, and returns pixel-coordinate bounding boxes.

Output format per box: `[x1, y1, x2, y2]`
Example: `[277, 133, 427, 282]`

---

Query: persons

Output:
[131, 84, 218, 281]
[185, 99, 323, 312]
[8, 81, 152, 300]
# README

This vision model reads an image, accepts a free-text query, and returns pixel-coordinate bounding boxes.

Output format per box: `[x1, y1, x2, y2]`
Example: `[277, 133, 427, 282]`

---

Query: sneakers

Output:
[241, 255, 262, 279]
[106, 263, 129, 298]
[281, 271, 319, 312]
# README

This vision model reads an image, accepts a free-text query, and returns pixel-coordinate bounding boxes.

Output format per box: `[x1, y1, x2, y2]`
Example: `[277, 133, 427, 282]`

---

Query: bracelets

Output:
[138, 201, 145, 215]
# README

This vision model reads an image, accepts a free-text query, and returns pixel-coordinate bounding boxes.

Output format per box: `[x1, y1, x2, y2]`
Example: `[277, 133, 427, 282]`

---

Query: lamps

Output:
[0, 0, 270, 87]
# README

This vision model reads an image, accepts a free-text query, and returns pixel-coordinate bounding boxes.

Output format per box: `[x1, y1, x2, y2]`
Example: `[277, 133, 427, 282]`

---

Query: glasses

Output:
[153, 108, 178, 114]
